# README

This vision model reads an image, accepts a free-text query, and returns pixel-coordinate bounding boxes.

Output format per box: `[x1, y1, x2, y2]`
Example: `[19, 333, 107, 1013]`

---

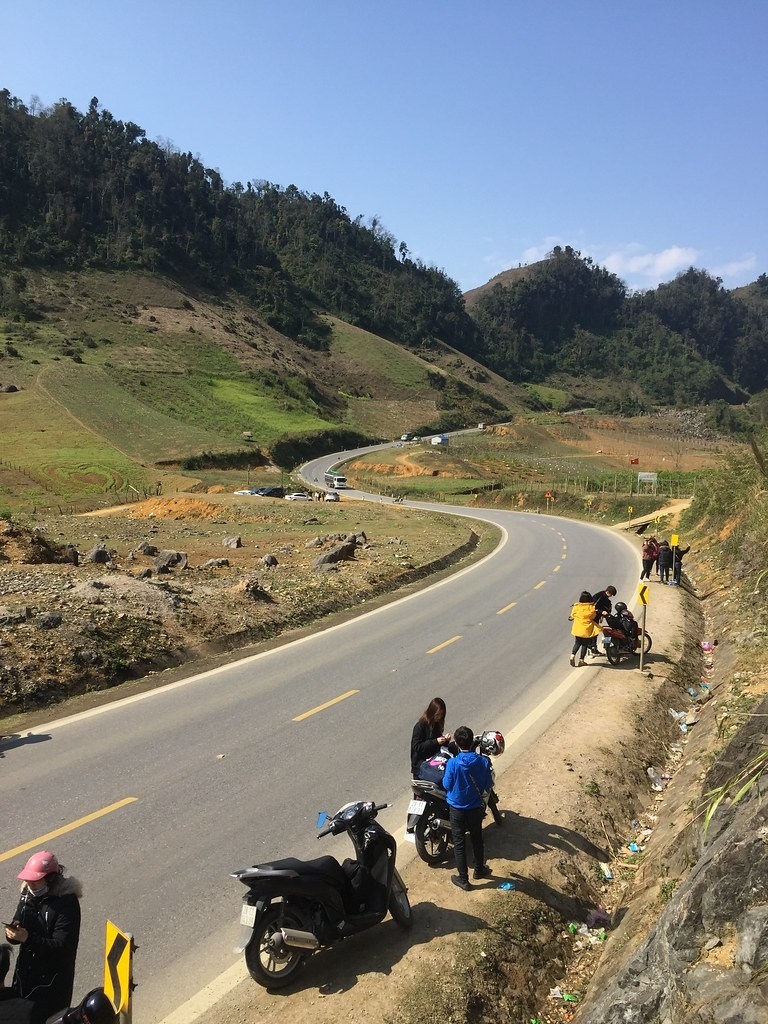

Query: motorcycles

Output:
[406, 730, 506, 865]
[600, 602, 653, 665]
[0, 987, 119, 1024]
[228, 799, 413, 990]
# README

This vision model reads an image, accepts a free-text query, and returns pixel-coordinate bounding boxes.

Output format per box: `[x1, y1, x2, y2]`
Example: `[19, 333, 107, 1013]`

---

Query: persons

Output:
[0, 943, 47, 1024]
[640, 538, 690, 585]
[403, 698, 451, 843]
[4, 851, 83, 1024]
[570, 592, 603, 667]
[442, 726, 494, 892]
[588, 586, 617, 657]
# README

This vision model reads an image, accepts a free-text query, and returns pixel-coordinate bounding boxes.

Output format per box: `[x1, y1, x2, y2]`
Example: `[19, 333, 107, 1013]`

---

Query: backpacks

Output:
[417, 749, 452, 783]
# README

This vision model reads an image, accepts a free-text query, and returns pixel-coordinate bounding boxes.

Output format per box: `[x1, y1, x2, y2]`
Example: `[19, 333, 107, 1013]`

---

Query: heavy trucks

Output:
[324, 469, 347, 490]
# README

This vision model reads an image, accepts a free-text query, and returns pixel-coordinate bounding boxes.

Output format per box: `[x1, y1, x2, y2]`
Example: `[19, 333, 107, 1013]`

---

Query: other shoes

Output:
[660, 580, 664, 585]
[665, 579, 679, 585]
[578, 659, 589, 666]
[655, 574, 661, 577]
[570, 654, 575, 667]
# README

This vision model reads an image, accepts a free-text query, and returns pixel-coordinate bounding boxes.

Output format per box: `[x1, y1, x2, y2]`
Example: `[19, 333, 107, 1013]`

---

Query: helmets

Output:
[76, 987, 116, 1024]
[17, 852, 62, 881]
[480, 731, 505, 756]
[615, 602, 627, 612]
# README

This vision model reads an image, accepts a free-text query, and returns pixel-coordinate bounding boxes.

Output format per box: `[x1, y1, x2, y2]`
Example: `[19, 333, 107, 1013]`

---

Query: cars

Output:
[325, 492, 339, 502]
[412, 436, 421, 441]
[284, 492, 313, 501]
[236, 486, 283, 498]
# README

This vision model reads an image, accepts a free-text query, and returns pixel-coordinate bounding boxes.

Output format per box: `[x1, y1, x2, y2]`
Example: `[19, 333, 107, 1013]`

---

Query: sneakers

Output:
[586, 650, 604, 657]
[640, 577, 651, 584]
[474, 868, 492, 879]
[451, 875, 472, 891]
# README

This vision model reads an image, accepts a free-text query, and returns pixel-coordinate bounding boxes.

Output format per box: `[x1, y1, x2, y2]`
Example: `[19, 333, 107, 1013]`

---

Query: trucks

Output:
[400, 431, 412, 442]
[478, 422, 487, 430]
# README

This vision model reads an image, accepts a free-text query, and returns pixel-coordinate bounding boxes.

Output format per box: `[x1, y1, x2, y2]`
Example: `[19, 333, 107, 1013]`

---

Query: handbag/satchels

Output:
[480, 802, 487, 819]
